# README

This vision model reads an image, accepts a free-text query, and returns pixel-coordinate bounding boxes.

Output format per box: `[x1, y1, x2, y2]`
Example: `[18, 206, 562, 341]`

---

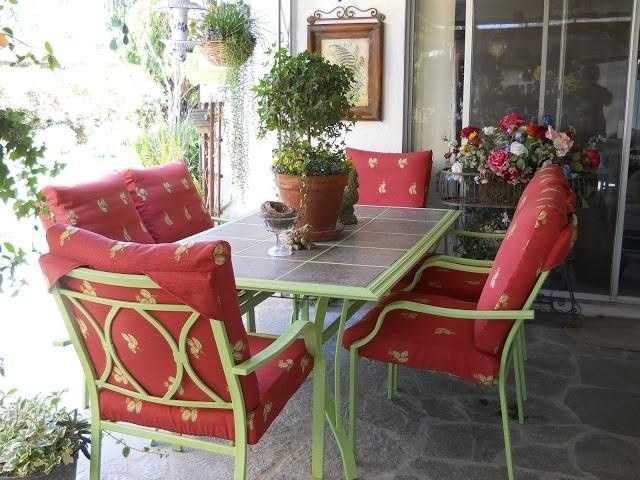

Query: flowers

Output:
[441, 113, 601, 185]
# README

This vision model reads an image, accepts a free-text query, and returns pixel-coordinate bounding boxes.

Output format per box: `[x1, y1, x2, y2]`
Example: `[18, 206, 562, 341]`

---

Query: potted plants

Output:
[0, 390, 170, 480]
[196, 3, 258, 82]
[251, 49, 356, 242]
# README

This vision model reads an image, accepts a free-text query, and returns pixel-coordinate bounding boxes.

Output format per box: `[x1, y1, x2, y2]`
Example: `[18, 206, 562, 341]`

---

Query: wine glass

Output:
[259, 214, 299, 258]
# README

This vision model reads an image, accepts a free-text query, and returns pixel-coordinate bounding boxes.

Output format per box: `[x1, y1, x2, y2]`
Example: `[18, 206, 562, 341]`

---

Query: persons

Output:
[565, 64, 612, 144]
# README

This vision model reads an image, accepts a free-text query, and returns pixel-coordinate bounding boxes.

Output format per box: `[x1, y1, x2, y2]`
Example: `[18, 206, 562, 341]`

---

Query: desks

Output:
[171, 206, 464, 480]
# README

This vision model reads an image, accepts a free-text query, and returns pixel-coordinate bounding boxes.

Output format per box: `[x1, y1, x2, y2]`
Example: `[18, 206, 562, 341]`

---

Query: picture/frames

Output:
[308, 23, 382, 122]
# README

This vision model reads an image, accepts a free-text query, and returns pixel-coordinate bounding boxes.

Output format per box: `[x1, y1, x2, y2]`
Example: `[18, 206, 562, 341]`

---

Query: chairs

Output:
[38, 223, 328, 480]
[299, 146, 433, 320]
[35, 171, 157, 407]
[122, 159, 255, 332]
[390, 165, 573, 403]
[343, 185, 578, 480]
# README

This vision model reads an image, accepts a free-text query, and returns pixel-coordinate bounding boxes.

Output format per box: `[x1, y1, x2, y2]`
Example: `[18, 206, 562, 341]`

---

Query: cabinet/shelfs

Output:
[439, 168, 585, 328]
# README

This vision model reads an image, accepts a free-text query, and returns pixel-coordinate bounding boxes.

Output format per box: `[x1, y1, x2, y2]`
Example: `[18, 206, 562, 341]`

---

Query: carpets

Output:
[564, 315, 640, 352]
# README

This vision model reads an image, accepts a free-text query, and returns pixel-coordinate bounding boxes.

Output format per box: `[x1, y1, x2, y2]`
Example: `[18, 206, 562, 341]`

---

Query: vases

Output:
[478, 174, 531, 204]
